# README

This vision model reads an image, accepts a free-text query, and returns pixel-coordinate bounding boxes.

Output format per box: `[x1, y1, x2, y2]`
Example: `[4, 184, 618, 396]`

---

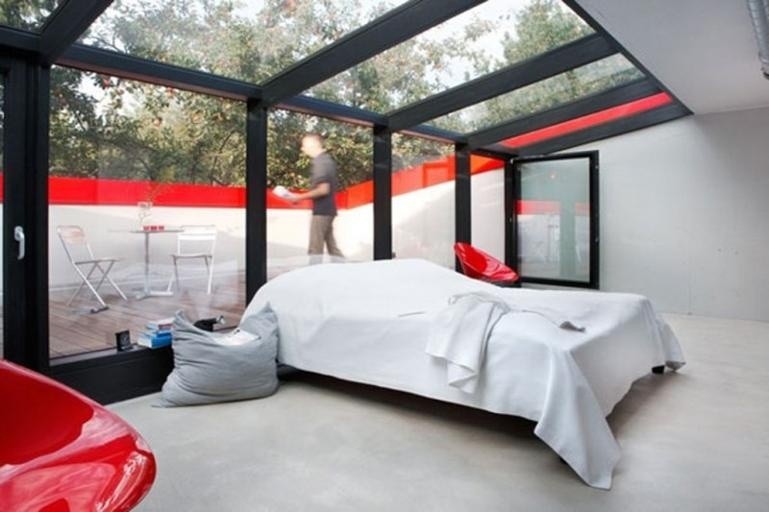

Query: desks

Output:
[109, 228, 184, 300]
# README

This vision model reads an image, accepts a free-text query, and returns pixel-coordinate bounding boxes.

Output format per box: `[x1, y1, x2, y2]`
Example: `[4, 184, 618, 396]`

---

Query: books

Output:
[136, 316, 173, 350]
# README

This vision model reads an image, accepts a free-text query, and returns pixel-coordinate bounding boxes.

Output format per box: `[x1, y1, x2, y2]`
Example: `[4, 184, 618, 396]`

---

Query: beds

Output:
[250, 257, 665, 464]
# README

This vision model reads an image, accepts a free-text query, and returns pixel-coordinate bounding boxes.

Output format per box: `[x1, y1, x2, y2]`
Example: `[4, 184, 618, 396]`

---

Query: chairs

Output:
[167, 225, 219, 295]
[0, 360, 156, 512]
[57, 225, 128, 309]
[453, 242, 520, 287]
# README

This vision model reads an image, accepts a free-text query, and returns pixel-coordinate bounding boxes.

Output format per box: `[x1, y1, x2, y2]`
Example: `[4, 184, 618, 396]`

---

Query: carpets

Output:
[100, 310, 767, 512]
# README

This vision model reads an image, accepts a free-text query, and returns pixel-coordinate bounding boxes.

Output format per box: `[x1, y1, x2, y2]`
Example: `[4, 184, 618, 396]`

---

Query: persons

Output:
[290, 134, 344, 266]
